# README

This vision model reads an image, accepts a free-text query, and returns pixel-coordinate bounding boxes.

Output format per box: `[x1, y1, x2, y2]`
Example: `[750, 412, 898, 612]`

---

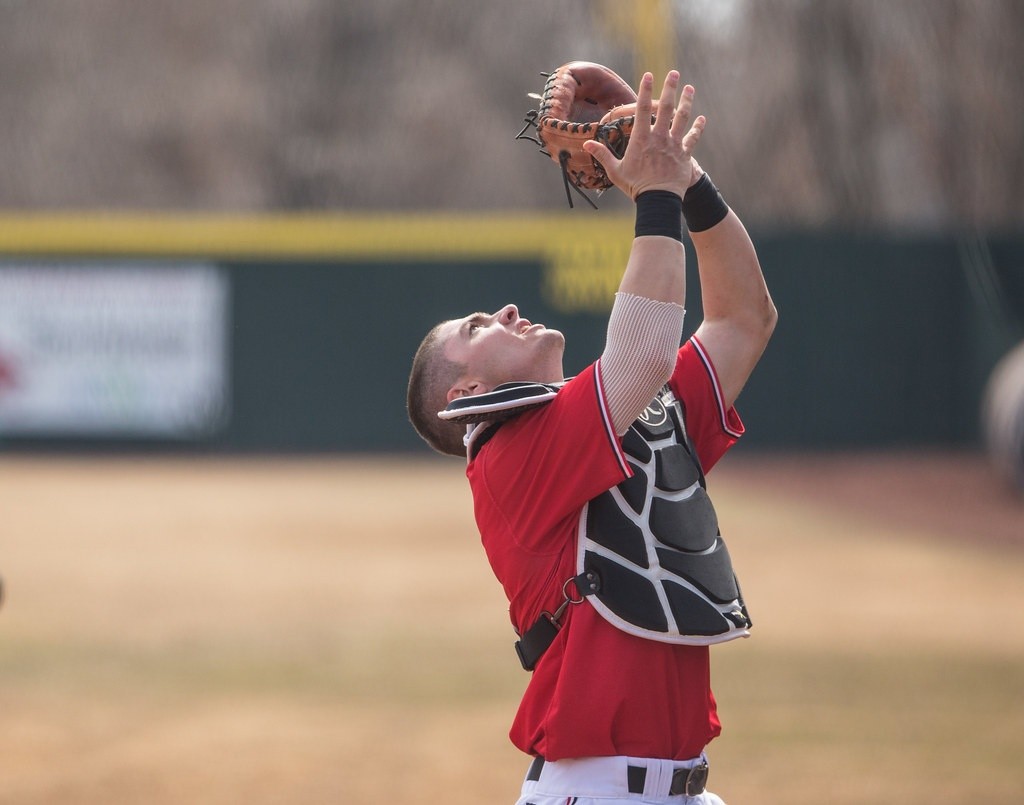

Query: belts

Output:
[529, 757, 709, 796]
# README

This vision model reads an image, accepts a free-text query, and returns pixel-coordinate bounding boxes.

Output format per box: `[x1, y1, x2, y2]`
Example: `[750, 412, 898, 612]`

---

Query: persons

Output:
[407, 63, 778, 805]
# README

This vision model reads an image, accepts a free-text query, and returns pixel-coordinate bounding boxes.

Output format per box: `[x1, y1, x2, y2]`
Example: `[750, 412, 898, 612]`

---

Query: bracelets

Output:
[631, 190, 685, 242]
[681, 173, 730, 233]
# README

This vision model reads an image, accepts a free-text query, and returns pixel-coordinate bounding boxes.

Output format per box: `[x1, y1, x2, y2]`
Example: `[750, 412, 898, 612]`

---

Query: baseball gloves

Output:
[535, 59, 675, 195]
